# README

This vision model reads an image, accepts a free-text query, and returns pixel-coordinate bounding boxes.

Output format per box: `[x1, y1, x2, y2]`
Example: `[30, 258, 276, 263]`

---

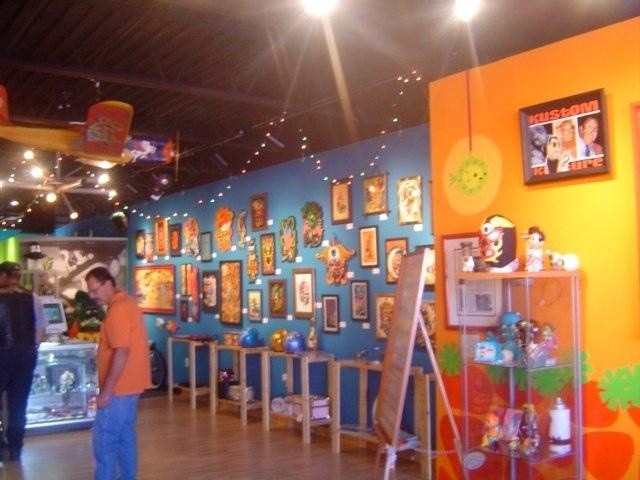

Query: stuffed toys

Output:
[477, 215, 519, 272]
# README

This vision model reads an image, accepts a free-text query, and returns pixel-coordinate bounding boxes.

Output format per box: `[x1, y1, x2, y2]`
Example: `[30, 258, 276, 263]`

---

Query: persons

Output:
[84, 267, 152, 480]
[452, 240, 480, 271]
[0, 262, 48, 457]
[529, 116, 601, 175]
[525, 225, 546, 272]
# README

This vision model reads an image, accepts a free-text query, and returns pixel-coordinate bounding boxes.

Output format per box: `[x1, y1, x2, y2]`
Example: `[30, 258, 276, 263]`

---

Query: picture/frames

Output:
[330, 180, 353, 224]
[385, 237, 409, 284]
[168, 224, 182, 257]
[350, 280, 370, 322]
[219, 261, 243, 325]
[293, 268, 315, 319]
[359, 225, 379, 267]
[320, 294, 340, 335]
[247, 289, 263, 323]
[180, 300, 188, 322]
[374, 293, 398, 341]
[269, 280, 289, 317]
[154, 218, 168, 256]
[135, 229, 145, 259]
[202, 270, 219, 314]
[396, 175, 422, 225]
[146, 233, 155, 262]
[362, 172, 389, 216]
[414, 299, 436, 350]
[518, 89, 610, 186]
[442, 231, 503, 332]
[193, 267, 200, 323]
[259, 233, 277, 275]
[250, 192, 267, 231]
[200, 232, 212, 262]
[132, 265, 176, 315]
[415, 244, 436, 292]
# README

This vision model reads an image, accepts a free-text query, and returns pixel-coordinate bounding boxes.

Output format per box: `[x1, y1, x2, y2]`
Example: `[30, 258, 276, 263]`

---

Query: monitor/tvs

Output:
[39, 295, 68, 334]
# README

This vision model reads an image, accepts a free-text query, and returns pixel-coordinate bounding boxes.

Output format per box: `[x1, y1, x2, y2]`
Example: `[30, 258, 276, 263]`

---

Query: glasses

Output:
[86, 280, 106, 298]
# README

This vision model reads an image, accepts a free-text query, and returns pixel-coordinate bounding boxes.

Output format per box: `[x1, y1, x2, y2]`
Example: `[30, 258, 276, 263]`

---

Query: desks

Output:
[167, 336, 217, 409]
[329, 358, 423, 469]
[260, 349, 334, 444]
[209, 341, 269, 425]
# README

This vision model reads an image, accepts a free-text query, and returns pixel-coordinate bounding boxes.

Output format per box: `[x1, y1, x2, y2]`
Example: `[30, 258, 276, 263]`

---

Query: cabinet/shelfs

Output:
[23, 341, 99, 436]
[457, 270, 584, 480]
